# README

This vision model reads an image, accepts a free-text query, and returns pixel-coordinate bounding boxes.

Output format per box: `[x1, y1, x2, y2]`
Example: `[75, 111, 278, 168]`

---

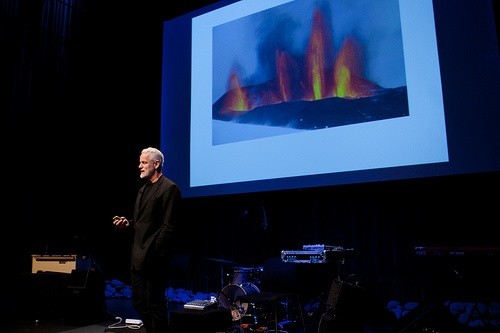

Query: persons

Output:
[111, 147, 186, 333]
